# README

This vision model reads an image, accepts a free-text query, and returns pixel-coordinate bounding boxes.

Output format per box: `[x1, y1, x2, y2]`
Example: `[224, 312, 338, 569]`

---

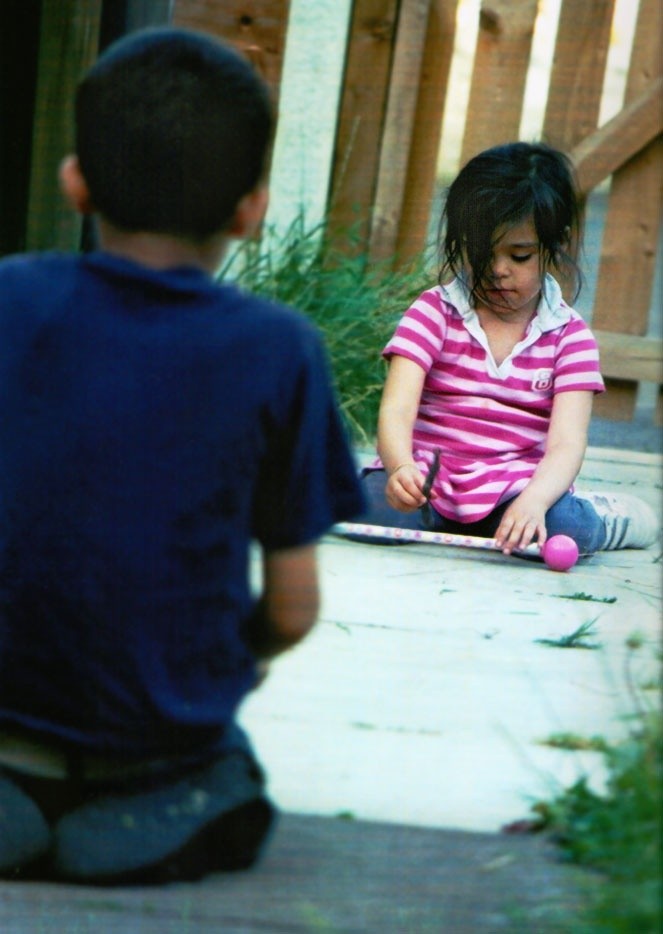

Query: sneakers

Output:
[572, 490, 657, 549]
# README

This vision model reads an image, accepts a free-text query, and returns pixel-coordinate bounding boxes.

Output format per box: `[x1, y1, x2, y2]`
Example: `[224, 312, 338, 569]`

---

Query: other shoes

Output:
[51, 757, 263, 878]
[0, 772, 49, 872]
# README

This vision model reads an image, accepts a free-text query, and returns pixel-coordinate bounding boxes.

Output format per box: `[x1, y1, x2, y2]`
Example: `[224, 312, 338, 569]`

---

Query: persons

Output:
[0, 27, 374, 887]
[342, 141, 659, 564]
